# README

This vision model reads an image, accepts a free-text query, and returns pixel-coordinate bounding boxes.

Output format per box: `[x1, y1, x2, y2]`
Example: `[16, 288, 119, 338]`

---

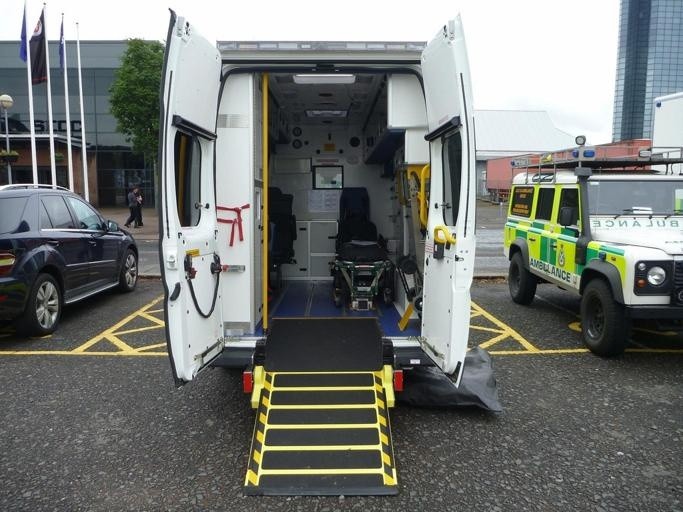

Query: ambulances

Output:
[147, 4, 476, 503]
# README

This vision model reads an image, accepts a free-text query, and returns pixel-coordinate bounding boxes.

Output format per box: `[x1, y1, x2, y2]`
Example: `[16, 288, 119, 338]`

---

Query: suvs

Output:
[0, 183, 139, 336]
[499, 137, 683, 354]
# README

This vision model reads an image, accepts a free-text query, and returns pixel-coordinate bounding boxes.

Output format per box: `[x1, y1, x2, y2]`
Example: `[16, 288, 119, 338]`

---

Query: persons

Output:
[124, 186, 143, 228]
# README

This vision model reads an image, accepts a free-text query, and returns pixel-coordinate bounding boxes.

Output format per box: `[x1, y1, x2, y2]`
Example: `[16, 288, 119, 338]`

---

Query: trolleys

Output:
[325, 219, 395, 312]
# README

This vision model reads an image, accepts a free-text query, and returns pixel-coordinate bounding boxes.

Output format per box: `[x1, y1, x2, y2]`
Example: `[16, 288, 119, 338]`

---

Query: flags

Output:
[29, 8, 47, 85]
[19, 10, 26, 63]
[59, 21, 64, 70]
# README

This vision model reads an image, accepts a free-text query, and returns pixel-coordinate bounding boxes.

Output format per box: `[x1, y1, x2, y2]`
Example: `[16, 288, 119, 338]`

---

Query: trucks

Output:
[648, 90, 682, 174]
[483, 138, 652, 202]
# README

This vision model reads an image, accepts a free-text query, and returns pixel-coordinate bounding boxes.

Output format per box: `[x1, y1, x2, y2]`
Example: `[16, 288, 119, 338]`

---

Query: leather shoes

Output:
[125, 221, 143, 228]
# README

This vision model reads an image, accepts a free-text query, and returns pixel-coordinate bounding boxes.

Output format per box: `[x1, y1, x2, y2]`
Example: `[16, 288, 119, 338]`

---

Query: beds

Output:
[327, 187, 395, 308]
[269, 185, 296, 270]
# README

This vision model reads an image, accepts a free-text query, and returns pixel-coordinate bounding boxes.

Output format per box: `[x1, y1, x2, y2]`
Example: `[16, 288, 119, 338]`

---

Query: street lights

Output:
[0, 92, 16, 184]
